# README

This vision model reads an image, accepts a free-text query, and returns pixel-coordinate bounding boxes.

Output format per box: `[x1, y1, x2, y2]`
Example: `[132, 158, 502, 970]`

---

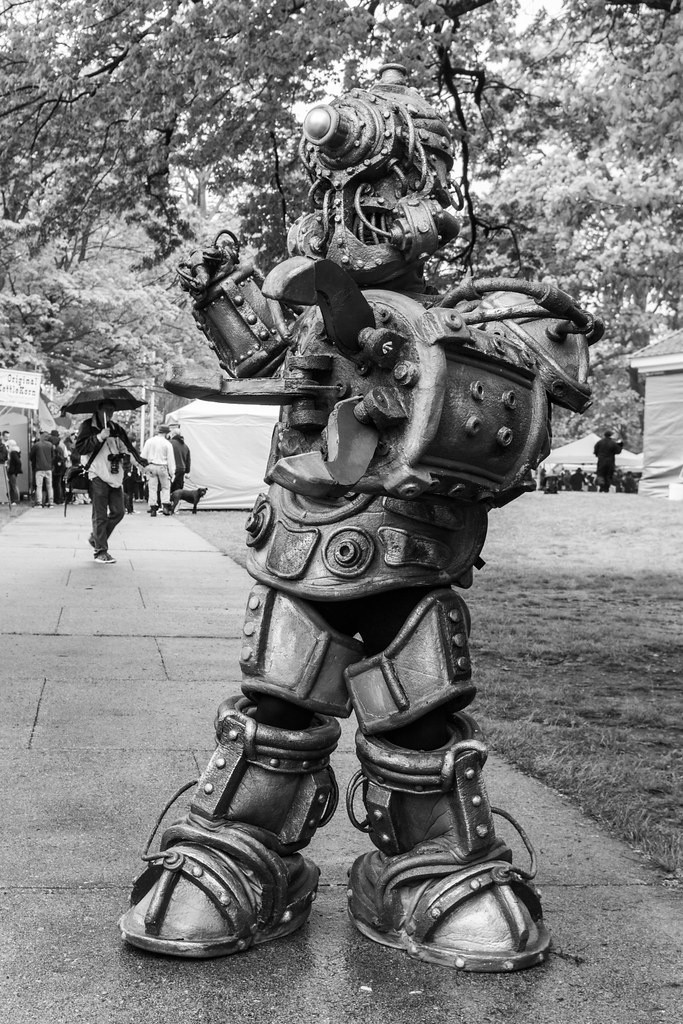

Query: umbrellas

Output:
[60, 384, 148, 432]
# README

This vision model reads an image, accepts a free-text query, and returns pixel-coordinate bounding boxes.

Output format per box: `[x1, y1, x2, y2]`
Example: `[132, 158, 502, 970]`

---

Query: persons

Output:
[75, 398, 150, 564]
[0, 424, 192, 516]
[593, 431, 624, 493]
[532, 466, 638, 495]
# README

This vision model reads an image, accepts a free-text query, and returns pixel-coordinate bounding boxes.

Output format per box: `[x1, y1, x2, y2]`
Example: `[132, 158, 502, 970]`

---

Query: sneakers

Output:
[94, 550, 116, 564]
[89, 537, 97, 547]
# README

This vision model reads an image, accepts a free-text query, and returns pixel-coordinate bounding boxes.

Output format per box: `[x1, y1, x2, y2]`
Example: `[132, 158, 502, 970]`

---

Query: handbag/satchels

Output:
[62, 463, 89, 490]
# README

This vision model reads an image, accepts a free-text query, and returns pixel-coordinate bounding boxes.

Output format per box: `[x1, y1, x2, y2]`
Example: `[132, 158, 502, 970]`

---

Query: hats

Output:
[48, 430, 59, 436]
[158, 425, 171, 432]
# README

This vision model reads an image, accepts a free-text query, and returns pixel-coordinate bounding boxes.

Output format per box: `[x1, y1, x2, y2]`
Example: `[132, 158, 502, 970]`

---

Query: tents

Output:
[636, 371, 683, 499]
[163, 398, 283, 513]
[0, 391, 76, 499]
[538, 432, 644, 490]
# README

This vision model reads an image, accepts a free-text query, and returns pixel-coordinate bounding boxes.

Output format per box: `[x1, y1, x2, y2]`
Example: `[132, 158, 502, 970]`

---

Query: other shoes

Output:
[151, 507, 156, 516]
[163, 506, 171, 515]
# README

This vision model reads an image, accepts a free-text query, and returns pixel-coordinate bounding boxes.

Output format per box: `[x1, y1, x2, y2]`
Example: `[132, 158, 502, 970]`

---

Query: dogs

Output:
[169, 487, 208, 515]
